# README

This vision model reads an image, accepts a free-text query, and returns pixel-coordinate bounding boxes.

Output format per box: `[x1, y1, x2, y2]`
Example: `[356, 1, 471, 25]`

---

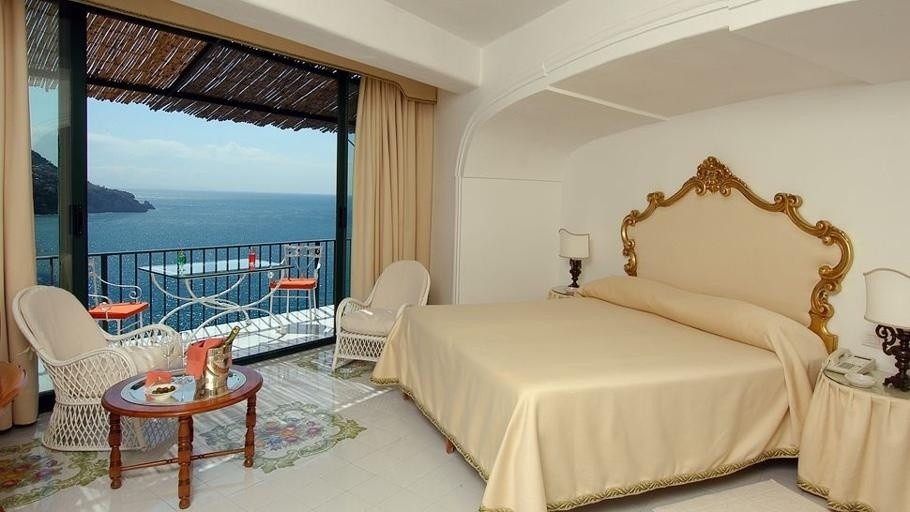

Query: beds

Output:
[368, 153, 856, 512]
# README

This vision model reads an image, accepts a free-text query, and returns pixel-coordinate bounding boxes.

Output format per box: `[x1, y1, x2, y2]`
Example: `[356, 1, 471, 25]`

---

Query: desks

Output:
[0, 360, 27, 408]
[136, 256, 298, 343]
[99, 366, 267, 510]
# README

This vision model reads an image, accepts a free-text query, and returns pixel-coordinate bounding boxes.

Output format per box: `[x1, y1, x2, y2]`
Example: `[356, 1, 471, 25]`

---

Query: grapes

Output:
[152, 386, 175, 394]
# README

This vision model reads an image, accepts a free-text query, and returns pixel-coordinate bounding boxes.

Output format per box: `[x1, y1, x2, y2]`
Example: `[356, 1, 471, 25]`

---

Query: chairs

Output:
[263, 242, 327, 330]
[7, 281, 193, 455]
[330, 252, 433, 376]
[87, 257, 152, 333]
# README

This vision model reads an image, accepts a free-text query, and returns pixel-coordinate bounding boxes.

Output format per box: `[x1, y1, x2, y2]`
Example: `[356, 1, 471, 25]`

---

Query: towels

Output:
[186, 336, 233, 379]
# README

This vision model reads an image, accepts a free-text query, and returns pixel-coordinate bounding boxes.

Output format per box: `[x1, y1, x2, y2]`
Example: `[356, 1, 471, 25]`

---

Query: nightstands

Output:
[792, 347, 910, 512]
[548, 281, 580, 301]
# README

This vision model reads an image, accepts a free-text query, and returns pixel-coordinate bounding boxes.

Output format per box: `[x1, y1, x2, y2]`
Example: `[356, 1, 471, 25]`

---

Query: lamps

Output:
[858, 262, 910, 393]
[557, 226, 592, 289]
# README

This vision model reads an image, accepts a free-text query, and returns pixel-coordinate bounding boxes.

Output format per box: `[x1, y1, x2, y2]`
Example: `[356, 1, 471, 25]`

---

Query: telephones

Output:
[822, 347, 875, 376]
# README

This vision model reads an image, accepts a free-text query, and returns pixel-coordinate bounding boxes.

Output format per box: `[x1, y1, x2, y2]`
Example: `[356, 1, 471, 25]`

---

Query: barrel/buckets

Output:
[187, 339, 233, 390]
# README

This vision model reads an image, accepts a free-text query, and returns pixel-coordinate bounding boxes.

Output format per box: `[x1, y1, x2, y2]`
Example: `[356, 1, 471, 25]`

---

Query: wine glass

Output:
[177, 254, 187, 273]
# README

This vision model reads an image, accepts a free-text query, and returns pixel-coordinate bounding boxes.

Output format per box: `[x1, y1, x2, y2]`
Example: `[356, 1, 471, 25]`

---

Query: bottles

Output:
[247, 246, 256, 271]
[223, 325, 240, 346]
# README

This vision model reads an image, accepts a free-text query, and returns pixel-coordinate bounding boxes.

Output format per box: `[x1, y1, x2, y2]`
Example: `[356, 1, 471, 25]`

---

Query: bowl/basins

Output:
[844, 372, 876, 388]
[145, 383, 181, 399]
